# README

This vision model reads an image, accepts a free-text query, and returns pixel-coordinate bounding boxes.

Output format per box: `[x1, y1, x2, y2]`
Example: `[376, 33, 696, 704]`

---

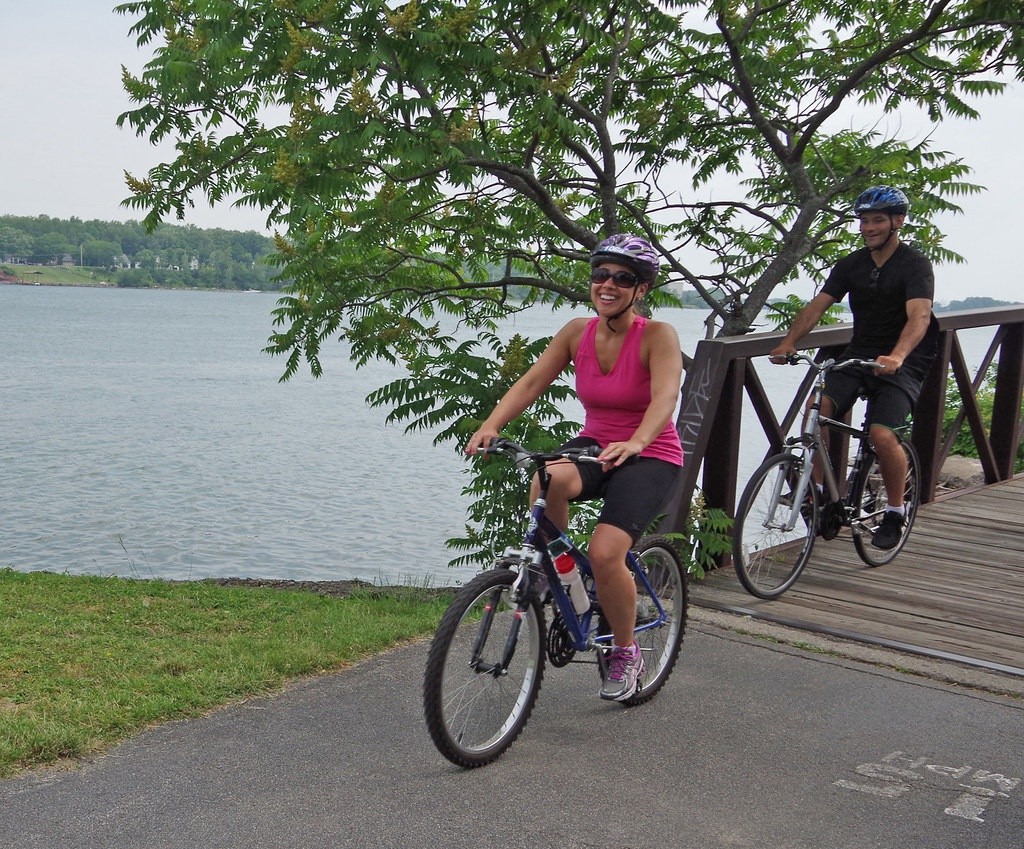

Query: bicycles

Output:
[420, 438, 690, 768]
[730, 353, 922, 601]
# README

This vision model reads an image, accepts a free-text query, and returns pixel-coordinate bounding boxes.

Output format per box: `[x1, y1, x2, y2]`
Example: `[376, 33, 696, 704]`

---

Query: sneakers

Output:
[778, 485, 822, 506]
[600, 640, 645, 701]
[871, 500, 914, 551]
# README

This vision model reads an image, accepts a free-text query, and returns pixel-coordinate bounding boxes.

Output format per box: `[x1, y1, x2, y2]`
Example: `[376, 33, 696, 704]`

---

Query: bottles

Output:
[553, 552, 590, 615]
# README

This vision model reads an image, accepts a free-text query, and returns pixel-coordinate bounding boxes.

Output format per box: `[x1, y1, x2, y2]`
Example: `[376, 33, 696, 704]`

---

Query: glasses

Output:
[592, 268, 641, 288]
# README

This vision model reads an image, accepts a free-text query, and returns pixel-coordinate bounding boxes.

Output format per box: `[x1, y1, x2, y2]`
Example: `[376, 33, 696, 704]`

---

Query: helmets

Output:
[854, 184, 909, 253]
[590, 233, 660, 333]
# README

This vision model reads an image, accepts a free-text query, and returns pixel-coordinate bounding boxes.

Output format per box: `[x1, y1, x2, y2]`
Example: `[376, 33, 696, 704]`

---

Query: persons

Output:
[465, 233, 682, 701]
[770, 184, 934, 551]
